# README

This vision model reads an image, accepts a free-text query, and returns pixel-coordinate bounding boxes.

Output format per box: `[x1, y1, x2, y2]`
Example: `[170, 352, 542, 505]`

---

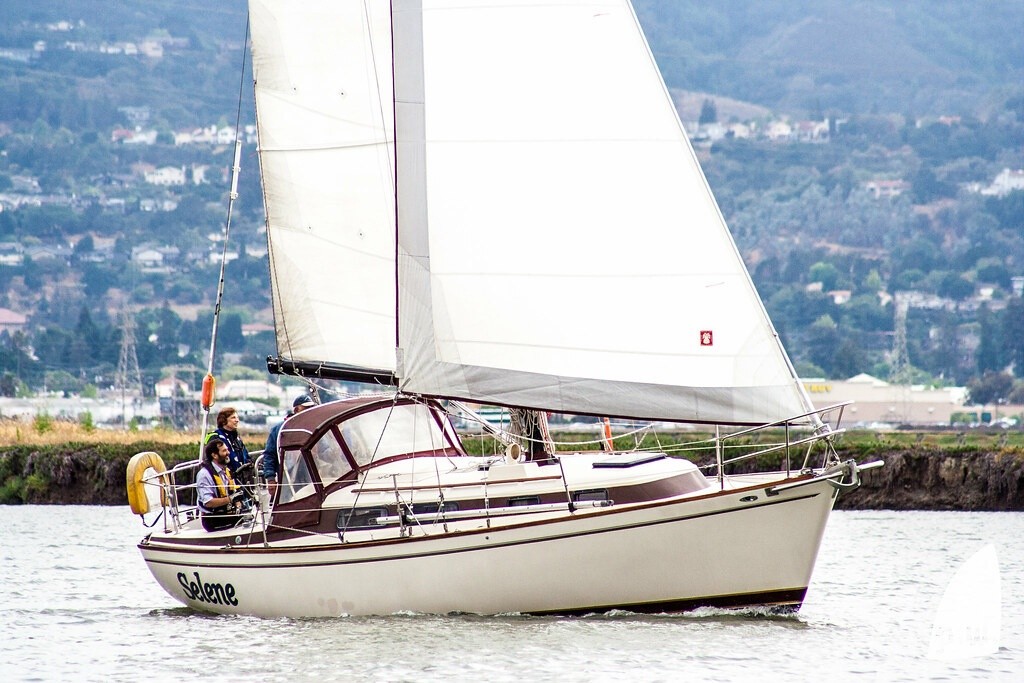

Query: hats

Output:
[293, 395, 316, 407]
[284, 410, 295, 418]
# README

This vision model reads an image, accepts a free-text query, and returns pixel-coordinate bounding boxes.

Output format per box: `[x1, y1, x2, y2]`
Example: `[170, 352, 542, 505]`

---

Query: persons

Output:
[263, 395, 328, 507]
[196, 439, 252, 532]
[206, 407, 260, 513]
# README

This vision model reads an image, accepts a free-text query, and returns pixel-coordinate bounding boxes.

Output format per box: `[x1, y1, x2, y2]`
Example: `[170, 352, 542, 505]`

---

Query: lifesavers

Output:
[127, 451, 171, 514]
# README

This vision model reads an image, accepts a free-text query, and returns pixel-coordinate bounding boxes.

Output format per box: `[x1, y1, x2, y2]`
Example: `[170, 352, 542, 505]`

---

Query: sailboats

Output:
[125, 0, 882, 623]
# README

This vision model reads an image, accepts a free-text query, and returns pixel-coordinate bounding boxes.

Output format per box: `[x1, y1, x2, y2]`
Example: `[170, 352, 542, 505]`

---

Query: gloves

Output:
[229, 489, 246, 503]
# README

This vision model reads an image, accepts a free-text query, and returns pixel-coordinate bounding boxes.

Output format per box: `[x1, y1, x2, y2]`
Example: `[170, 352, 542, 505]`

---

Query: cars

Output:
[855, 417, 1016, 435]
